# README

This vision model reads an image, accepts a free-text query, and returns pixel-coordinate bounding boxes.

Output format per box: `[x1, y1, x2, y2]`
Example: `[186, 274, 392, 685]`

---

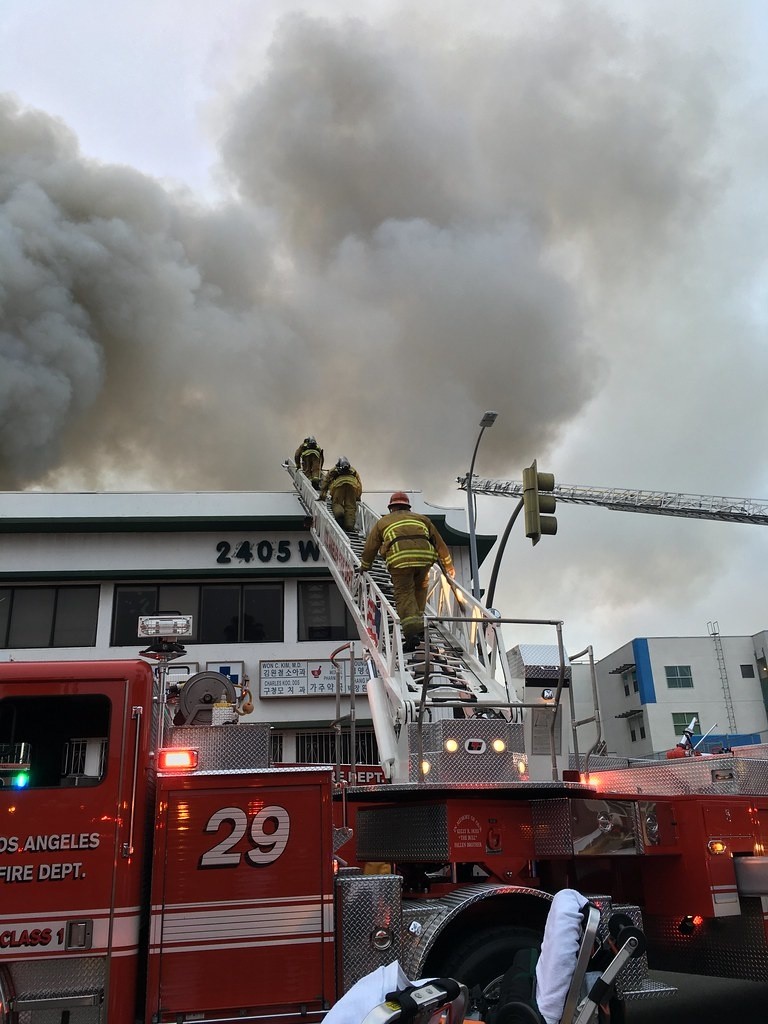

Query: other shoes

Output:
[311, 480, 320, 490]
[345, 526, 353, 532]
[335, 513, 344, 529]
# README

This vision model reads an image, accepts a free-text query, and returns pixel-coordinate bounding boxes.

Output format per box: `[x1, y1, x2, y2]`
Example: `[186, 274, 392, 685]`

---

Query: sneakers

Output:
[402, 635, 420, 653]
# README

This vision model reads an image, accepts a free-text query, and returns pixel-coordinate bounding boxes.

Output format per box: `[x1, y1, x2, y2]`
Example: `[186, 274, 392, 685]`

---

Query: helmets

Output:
[388, 492, 412, 509]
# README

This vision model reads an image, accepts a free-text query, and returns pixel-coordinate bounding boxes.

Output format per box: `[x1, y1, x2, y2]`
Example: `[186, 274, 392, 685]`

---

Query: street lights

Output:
[468, 411, 498, 601]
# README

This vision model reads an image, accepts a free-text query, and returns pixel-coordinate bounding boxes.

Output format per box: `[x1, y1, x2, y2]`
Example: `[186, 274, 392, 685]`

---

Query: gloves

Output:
[356, 497, 361, 501]
[296, 467, 301, 472]
[315, 497, 325, 502]
[360, 565, 372, 575]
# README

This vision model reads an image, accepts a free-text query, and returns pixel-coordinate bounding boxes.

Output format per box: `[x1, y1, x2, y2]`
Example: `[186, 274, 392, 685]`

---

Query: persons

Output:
[314, 456, 362, 532]
[295, 437, 324, 489]
[360, 491, 455, 654]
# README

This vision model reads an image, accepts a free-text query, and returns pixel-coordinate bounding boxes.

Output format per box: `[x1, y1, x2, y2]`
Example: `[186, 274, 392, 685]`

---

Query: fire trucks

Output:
[0, 458, 768, 1024]
